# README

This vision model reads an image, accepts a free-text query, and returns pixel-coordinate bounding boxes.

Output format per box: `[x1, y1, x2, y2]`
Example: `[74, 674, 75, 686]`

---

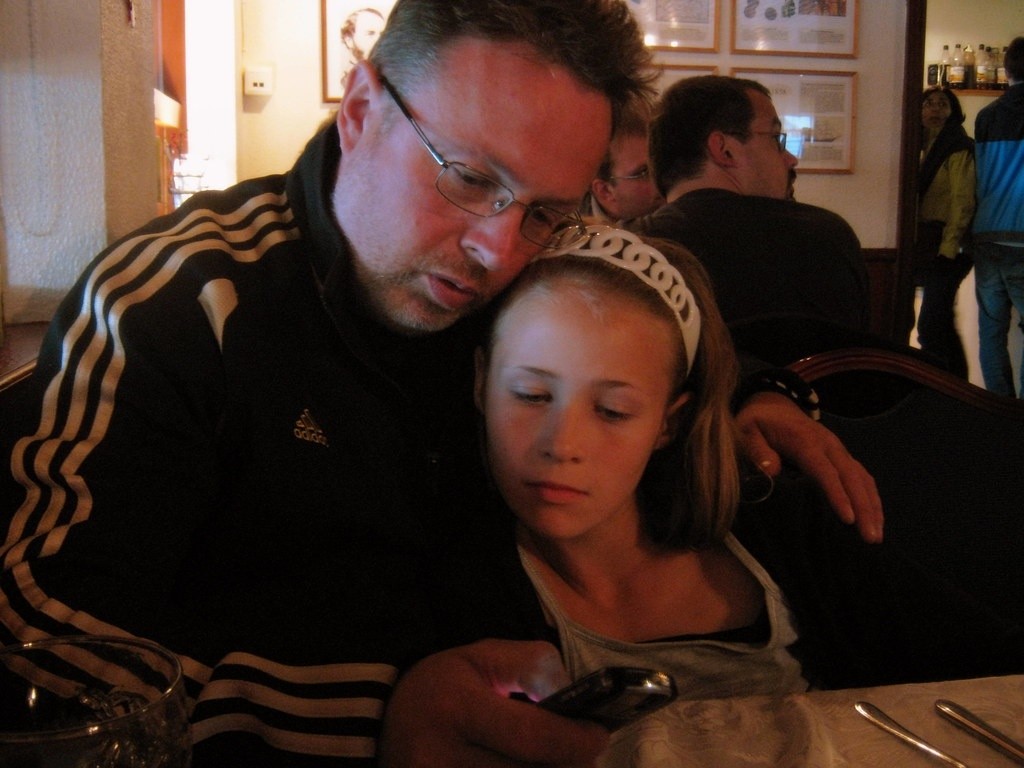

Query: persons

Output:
[913, 84, 975, 381]
[578, 105, 659, 226]
[468, 218, 837, 768]
[644, 75, 864, 367]
[974, 36, 1024, 398]
[0, 0, 885, 768]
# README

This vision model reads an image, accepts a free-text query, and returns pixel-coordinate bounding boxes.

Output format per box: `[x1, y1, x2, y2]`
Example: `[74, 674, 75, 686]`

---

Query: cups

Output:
[0, 635, 193, 768]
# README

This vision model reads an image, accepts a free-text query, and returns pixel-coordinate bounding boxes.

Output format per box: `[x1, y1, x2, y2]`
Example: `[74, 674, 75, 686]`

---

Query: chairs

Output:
[731, 343, 1024, 690]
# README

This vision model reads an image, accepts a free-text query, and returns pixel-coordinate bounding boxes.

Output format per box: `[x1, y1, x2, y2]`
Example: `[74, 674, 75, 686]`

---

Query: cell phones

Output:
[534, 665, 678, 733]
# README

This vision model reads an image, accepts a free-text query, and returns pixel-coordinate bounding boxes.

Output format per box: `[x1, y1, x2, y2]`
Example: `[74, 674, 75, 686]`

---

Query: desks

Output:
[593, 674, 1024, 768]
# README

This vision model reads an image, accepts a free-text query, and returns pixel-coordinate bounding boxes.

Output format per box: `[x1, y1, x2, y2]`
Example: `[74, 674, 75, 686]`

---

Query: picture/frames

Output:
[619, 0, 722, 54]
[318, 0, 404, 104]
[622, 62, 720, 119]
[728, 0, 860, 61]
[728, 67, 862, 176]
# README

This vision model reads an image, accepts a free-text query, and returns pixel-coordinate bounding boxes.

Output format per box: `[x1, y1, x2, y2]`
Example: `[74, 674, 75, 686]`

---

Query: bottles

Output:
[939, 44, 1009, 90]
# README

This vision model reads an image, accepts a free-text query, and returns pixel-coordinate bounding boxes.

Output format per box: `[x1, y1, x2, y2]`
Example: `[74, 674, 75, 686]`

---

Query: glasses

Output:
[609, 169, 648, 182]
[747, 132, 786, 150]
[377, 72, 586, 247]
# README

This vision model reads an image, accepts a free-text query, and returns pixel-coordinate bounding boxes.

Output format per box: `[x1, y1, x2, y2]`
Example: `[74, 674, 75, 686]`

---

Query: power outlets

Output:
[242, 67, 276, 97]
